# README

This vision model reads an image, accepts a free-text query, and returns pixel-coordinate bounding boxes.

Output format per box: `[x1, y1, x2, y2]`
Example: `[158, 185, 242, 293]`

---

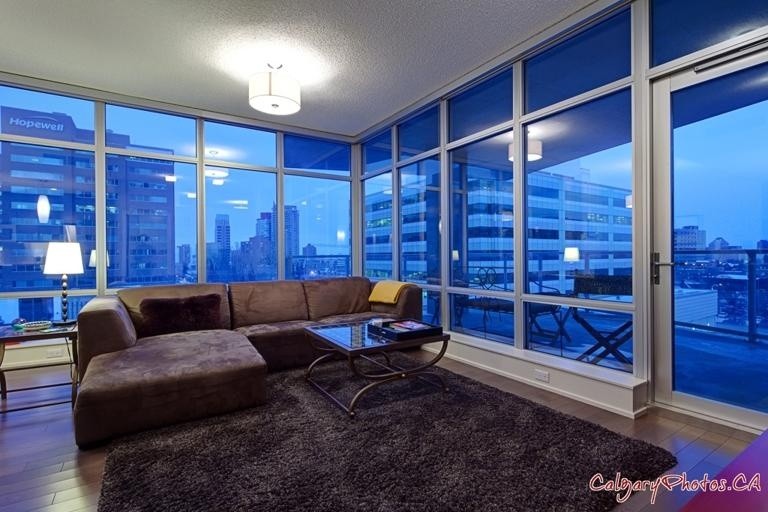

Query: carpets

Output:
[93, 344, 680, 512]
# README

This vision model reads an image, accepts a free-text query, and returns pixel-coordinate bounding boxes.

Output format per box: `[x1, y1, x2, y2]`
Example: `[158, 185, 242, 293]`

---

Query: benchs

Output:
[424, 266, 574, 347]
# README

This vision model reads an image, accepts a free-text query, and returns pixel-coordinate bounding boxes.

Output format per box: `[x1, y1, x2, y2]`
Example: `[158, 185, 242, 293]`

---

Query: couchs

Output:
[69, 277, 422, 452]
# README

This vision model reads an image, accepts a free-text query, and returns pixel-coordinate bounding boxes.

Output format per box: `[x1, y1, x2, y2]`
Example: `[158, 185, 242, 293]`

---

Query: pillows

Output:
[136, 292, 224, 338]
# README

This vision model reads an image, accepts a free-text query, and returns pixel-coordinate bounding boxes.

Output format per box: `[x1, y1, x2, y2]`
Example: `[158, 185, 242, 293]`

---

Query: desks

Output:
[0, 319, 78, 409]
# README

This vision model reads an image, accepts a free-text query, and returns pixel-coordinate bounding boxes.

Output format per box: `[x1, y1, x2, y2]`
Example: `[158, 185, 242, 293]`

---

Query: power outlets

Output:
[532, 368, 549, 384]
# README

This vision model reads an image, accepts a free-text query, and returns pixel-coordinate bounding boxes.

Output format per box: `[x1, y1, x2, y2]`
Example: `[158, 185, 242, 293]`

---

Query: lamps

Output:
[40, 241, 87, 326]
[624, 193, 632, 209]
[203, 148, 228, 178]
[247, 62, 303, 116]
[563, 247, 581, 275]
[36, 194, 111, 267]
[506, 127, 545, 164]
[451, 248, 460, 282]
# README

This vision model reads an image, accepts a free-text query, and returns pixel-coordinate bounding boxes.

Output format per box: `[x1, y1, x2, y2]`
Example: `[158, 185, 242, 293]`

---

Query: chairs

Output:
[568, 271, 654, 372]
